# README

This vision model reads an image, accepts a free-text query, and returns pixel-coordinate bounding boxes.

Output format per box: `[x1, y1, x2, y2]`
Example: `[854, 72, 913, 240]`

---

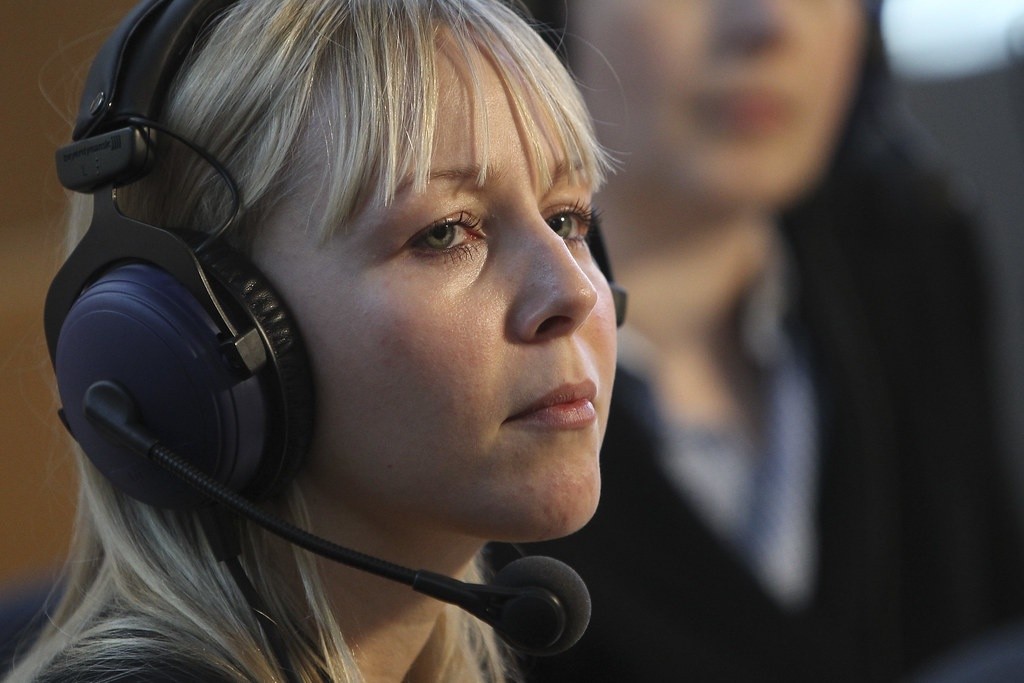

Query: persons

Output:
[482, 0, 1023, 683]
[0, 1, 618, 683]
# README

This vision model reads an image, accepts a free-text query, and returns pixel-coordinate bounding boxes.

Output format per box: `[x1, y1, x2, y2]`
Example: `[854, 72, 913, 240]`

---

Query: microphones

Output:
[149, 450, 592, 658]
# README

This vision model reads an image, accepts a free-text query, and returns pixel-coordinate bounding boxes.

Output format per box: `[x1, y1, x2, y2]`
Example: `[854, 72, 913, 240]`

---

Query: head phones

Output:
[41, 0, 627, 513]
